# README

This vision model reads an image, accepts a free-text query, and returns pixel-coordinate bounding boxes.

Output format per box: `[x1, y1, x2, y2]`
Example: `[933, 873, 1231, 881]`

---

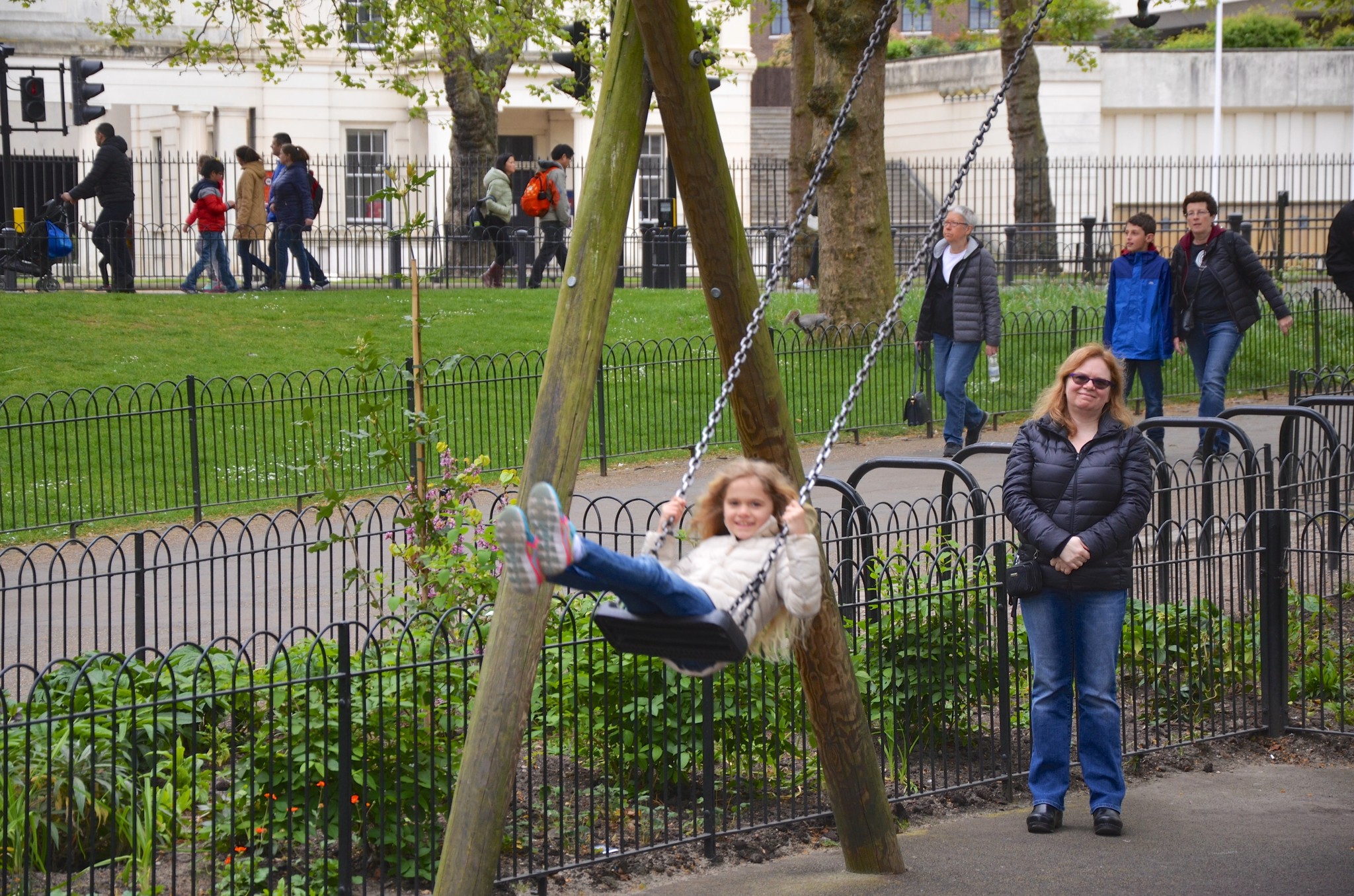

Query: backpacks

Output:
[305, 170, 323, 219]
[465, 196, 496, 241]
[520, 166, 560, 218]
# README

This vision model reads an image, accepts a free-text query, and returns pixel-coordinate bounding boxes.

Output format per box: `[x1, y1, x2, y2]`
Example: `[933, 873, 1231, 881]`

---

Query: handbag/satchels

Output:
[902, 390, 927, 426]
[1178, 304, 1194, 342]
[46, 220, 72, 258]
[1005, 560, 1042, 598]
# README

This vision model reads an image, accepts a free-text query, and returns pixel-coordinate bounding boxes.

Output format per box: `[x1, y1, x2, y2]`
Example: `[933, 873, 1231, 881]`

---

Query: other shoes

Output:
[93, 284, 136, 293]
[1212, 447, 1230, 457]
[1148, 442, 1164, 458]
[1193, 446, 1217, 462]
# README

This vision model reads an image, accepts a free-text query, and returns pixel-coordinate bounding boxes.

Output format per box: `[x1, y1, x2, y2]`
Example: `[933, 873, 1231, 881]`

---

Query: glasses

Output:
[942, 220, 969, 227]
[1067, 373, 1115, 390]
[1185, 209, 1210, 218]
[566, 155, 572, 162]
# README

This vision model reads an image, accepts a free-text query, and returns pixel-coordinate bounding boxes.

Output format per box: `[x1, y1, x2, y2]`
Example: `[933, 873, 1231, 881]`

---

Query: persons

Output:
[1325, 199, 1354, 309]
[527, 144, 572, 288]
[1102, 212, 1175, 459]
[998, 342, 1153, 836]
[256, 132, 330, 291]
[479, 153, 515, 289]
[233, 146, 282, 292]
[495, 460, 824, 679]
[179, 154, 246, 294]
[914, 205, 1002, 456]
[1170, 191, 1294, 463]
[60, 122, 136, 293]
[268, 144, 314, 291]
[795, 201, 818, 289]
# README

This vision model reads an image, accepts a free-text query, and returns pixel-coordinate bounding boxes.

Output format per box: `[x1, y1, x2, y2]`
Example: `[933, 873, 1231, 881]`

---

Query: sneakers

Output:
[181, 272, 330, 295]
[966, 411, 990, 446]
[495, 507, 543, 594]
[943, 441, 962, 457]
[526, 481, 576, 577]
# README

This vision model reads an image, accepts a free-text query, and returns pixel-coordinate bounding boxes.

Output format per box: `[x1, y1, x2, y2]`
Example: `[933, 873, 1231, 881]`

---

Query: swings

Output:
[588, 0, 1060, 663]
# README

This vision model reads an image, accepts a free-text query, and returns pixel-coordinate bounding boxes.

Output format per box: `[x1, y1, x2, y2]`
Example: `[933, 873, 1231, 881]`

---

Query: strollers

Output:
[0, 193, 72, 294]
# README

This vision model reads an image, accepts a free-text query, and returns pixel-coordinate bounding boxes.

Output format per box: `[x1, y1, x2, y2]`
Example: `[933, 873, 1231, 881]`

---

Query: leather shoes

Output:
[1093, 806, 1123, 836]
[1026, 803, 1063, 833]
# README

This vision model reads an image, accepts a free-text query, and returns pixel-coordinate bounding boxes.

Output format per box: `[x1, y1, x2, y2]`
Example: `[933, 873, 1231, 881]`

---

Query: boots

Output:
[479, 261, 504, 288]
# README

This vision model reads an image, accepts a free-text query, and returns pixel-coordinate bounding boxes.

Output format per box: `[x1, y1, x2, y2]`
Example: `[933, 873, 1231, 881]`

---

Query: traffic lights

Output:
[19, 76, 47, 123]
[697, 25, 722, 90]
[70, 54, 106, 126]
[551, 19, 594, 98]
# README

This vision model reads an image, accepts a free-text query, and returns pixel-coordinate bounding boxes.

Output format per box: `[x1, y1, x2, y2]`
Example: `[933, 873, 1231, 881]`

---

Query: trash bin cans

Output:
[638, 221, 659, 287]
[652, 226, 688, 289]
